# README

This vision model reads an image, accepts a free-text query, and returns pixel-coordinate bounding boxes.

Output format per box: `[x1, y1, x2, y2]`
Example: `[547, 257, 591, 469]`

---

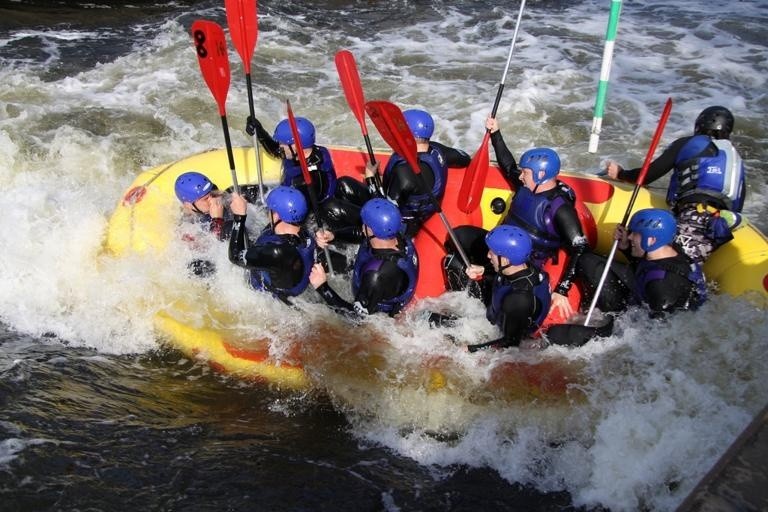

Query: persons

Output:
[309, 195, 417, 325]
[245, 114, 334, 225]
[548, 210, 691, 348]
[174, 172, 259, 280]
[431, 226, 548, 352]
[326, 110, 470, 241]
[604, 105, 746, 310]
[228, 185, 315, 302]
[446, 116, 586, 319]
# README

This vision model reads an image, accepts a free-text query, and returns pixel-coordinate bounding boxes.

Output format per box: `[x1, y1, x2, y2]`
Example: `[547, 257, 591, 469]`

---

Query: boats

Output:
[95, 134, 767, 436]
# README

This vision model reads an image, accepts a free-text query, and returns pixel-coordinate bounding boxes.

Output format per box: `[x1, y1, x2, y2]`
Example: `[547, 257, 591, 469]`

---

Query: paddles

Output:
[191, 20, 240, 194]
[335, 50, 386, 196]
[224, 0, 264, 202]
[457, 0, 527, 215]
[363, 100, 482, 281]
[584, 97, 671, 325]
[286, 98, 337, 275]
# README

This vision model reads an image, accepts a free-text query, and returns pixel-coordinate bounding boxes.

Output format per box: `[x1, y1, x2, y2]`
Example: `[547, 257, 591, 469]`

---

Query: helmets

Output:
[273, 118, 315, 148]
[265, 187, 307, 223]
[629, 208, 677, 252]
[403, 109, 434, 139]
[485, 224, 532, 265]
[695, 106, 733, 133]
[360, 198, 402, 239]
[519, 148, 560, 184]
[175, 172, 213, 203]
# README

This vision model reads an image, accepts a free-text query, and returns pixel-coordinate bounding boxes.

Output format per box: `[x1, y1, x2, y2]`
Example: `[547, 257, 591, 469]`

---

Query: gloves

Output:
[246, 117, 265, 141]
